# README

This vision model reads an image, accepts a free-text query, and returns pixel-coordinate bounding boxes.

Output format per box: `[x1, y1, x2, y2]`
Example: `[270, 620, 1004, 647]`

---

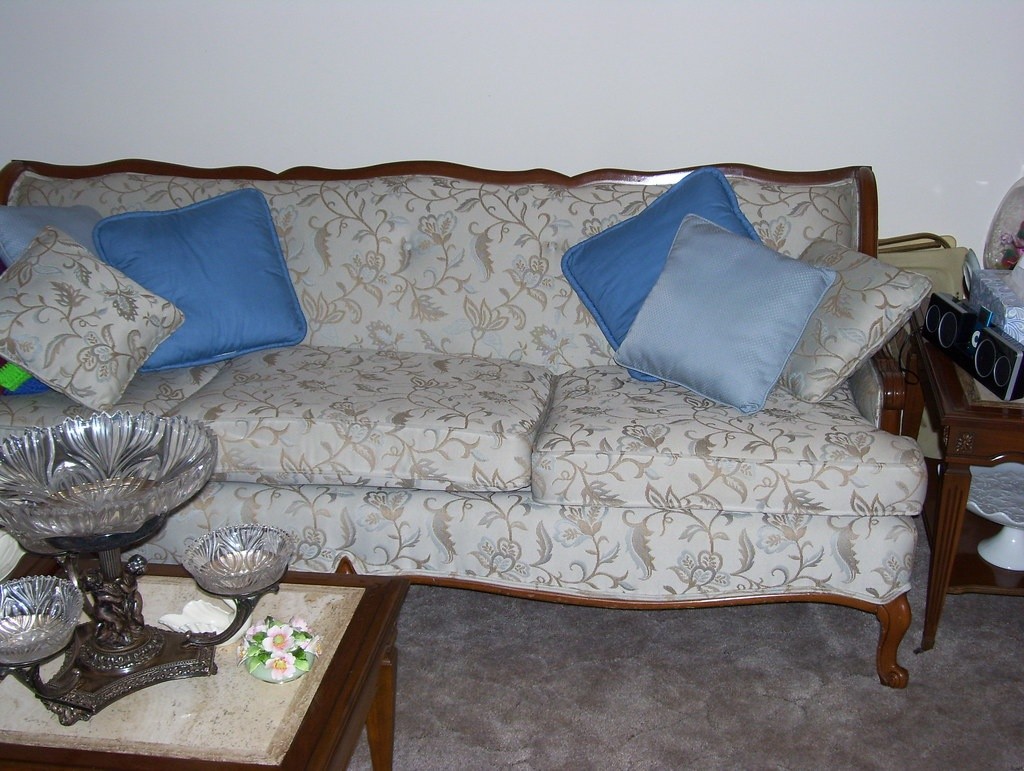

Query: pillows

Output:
[91, 188, 307, 373]
[776, 237, 933, 404]
[612, 213, 838, 415]
[560, 166, 763, 382]
[0, 206, 104, 268]
[0, 224, 185, 416]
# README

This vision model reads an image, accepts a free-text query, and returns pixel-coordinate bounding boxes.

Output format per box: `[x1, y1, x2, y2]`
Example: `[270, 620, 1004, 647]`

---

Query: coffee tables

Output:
[914, 305, 1024, 652]
[1, 551, 410, 771]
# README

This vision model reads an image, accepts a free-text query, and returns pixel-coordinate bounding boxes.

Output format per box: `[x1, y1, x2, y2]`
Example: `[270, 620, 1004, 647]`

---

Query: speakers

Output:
[921, 293, 1024, 401]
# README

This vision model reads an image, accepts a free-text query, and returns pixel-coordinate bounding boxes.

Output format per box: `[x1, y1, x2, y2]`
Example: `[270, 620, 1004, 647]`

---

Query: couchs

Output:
[0, 156, 927, 688]
